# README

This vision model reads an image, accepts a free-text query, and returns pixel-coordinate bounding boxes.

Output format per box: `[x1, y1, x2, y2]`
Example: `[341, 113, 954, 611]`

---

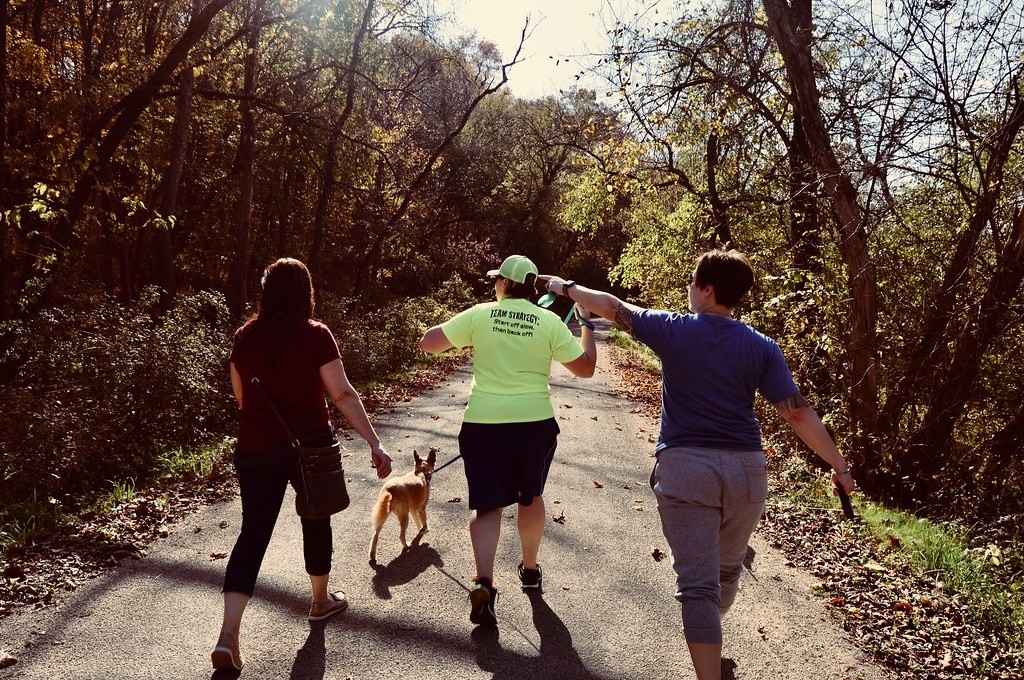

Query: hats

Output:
[487, 255, 538, 286]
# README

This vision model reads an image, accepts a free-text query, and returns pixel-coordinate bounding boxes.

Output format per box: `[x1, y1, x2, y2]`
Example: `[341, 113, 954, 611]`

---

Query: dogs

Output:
[368, 450, 436, 560]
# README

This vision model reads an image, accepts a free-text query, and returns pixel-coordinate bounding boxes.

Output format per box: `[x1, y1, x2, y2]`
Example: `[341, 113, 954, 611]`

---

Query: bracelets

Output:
[580, 323, 584, 326]
[837, 471, 850, 476]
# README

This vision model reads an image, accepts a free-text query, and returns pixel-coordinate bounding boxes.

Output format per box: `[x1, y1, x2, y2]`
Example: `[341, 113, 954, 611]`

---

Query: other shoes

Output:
[309, 590, 349, 620]
[211, 633, 245, 671]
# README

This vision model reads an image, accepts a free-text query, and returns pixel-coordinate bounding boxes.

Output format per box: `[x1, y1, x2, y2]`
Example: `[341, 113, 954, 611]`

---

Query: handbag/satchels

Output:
[295, 441, 350, 519]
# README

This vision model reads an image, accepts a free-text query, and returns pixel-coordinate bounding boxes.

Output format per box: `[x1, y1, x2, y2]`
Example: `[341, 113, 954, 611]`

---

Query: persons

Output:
[538, 250, 855, 680]
[211, 258, 392, 669]
[419, 255, 597, 623]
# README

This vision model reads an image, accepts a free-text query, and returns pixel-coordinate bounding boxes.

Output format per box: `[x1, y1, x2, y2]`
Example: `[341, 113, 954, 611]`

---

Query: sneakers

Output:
[517, 561, 543, 588]
[467, 577, 500, 625]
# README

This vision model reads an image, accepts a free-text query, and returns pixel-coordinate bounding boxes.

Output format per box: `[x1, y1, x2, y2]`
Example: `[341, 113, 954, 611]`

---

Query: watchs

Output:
[562, 280, 576, 296]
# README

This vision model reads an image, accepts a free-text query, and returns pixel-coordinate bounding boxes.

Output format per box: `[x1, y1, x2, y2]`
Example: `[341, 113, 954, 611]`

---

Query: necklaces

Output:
[703, 310, 730, 317]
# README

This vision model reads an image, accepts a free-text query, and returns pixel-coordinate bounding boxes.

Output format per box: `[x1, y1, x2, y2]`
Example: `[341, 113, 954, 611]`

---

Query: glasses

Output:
[688, 274, 700, 284]
[537, 291, 556, 307]
